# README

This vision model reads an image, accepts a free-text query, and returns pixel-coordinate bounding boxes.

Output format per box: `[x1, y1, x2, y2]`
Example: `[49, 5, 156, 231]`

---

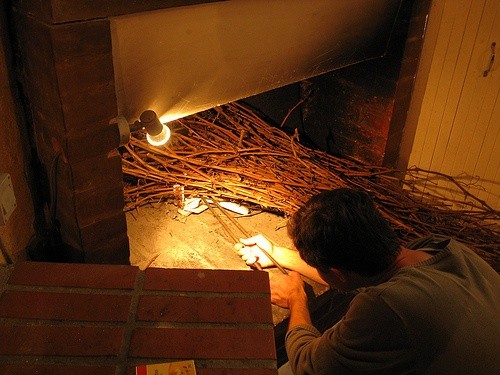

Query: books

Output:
[135, 359, 196, 375]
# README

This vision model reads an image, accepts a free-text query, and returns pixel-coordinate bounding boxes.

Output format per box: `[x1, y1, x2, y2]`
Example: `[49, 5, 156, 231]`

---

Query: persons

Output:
[233, 187, 500, 375]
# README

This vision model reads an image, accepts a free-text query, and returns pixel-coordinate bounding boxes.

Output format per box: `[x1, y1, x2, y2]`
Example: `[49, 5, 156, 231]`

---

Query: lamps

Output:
[117, 110, 172, 148]
